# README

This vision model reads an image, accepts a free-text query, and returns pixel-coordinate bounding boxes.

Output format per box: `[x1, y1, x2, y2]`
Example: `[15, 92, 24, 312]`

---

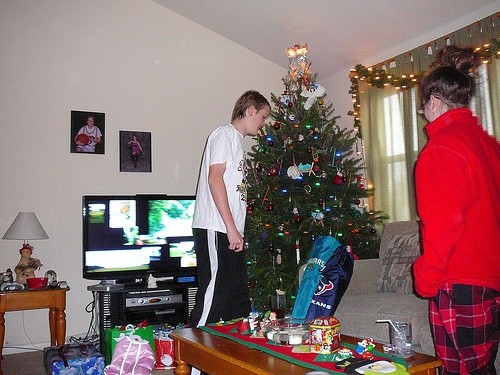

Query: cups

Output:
[389, 322, 412, 358]
[270, 294, 286, 319]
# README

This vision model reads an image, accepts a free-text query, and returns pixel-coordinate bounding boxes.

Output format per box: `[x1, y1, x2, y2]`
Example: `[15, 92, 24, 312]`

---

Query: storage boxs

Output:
[43, 343, 106, 375]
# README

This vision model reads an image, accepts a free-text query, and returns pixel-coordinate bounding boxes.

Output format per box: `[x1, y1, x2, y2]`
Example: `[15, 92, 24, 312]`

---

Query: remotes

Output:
[59, 281, 67, 289]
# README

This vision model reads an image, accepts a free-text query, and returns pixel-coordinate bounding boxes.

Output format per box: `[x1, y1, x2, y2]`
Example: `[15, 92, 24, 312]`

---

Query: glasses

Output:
[417, 96, 440, 115]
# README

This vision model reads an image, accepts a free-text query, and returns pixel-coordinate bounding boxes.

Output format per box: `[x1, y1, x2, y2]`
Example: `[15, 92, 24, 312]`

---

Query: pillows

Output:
[376, 234, 424, 297]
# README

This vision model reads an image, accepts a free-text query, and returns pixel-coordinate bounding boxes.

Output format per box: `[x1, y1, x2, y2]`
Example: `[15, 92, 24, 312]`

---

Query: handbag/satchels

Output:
[105, 324, 154, 366]
[154, 336, 177, 370]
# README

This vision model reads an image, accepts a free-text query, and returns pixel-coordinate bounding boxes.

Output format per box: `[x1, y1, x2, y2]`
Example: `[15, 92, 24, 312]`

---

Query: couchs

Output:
[298, 220, 500, 375]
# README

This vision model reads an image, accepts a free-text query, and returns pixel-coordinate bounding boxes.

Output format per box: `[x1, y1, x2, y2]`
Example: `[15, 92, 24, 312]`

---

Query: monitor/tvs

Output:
[82, 194, 200, 289]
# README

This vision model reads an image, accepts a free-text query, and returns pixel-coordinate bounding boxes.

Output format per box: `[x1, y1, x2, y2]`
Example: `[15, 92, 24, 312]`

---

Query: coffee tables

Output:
[169, 318, 442, 375]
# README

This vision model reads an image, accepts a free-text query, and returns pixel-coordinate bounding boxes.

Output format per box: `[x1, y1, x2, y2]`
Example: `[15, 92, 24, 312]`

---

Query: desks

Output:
[86, 280, 200, 355]
[0, 288, 67, 360]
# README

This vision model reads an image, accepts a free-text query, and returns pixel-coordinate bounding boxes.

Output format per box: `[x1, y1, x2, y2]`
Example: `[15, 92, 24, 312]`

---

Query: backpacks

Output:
[103, 335, 156, 375]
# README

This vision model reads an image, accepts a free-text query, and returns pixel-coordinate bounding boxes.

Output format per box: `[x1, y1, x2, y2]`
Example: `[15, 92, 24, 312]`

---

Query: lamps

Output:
[2, 212, 49, 244]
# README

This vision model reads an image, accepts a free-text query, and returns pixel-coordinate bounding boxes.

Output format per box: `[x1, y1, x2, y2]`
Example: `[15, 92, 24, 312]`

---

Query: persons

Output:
[412, 66, 500, 375]
[128, 135, 144, 167]
[190, 90, 271, 375]
[75, 115, 103, 152]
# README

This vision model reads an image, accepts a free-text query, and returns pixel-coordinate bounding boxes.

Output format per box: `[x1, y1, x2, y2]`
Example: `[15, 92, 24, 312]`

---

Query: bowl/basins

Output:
[26, 277, 49, 289]
